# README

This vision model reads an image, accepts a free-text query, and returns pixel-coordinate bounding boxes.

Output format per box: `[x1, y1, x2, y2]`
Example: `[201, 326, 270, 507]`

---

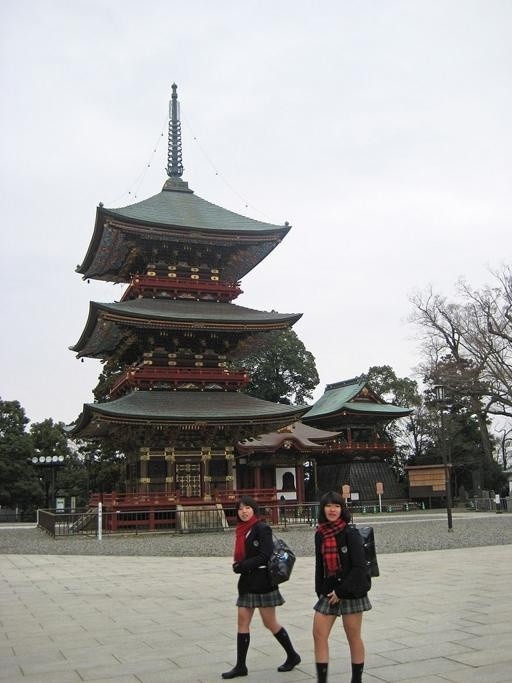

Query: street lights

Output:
[26, 454, 71, 510]
[435, 383, 454, 534]
[85, 452, 105, 540]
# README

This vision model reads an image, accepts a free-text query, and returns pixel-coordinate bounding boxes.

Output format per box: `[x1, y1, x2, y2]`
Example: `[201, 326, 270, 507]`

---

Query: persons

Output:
[308, 489, 374, 682]
[278, 494, 287, 522]
[219, 494, 302, 680]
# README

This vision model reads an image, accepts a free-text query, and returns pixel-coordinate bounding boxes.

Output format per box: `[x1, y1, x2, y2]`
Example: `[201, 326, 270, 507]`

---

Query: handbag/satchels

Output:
[355, 526, 380, 577]
[268, 538, 295, 584]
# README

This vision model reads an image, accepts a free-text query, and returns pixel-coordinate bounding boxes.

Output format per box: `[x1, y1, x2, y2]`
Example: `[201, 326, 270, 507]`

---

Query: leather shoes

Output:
[278, 654, 301, 671]
[221, 666, 247, 679]
